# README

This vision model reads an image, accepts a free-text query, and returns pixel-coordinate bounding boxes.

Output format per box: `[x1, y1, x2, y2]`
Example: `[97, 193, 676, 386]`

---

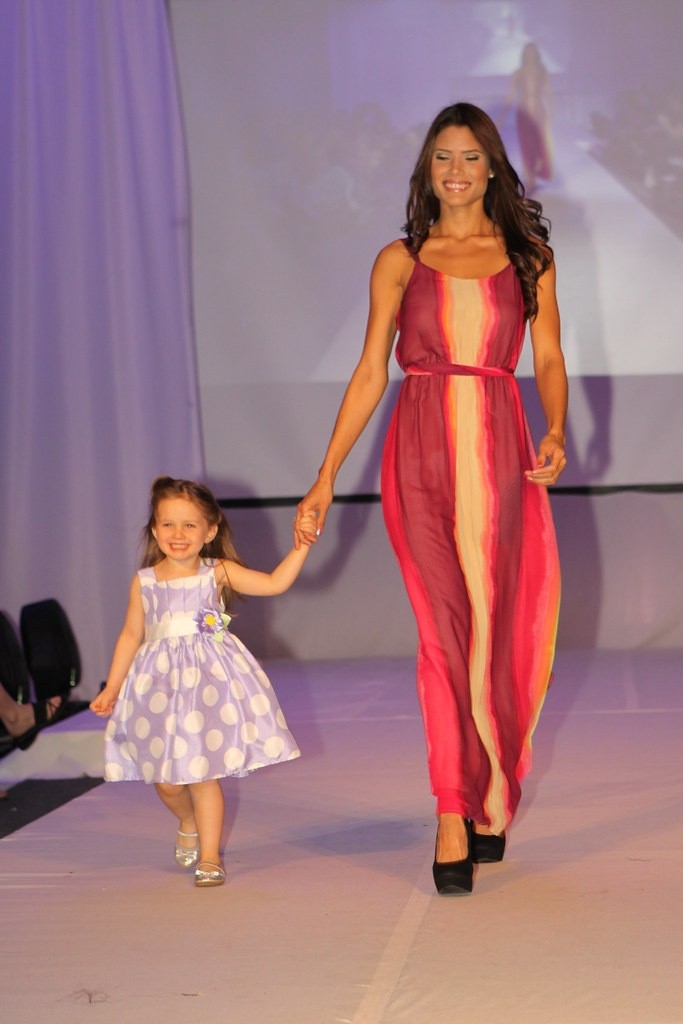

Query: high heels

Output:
[433, 827, 472, 894]
[470, 813, 506, 865]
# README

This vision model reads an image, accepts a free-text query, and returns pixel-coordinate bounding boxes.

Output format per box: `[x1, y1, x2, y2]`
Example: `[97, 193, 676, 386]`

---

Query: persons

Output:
[0, 679, 68, 802]
[89, 477, 318, 887]
[298, 103, 568, 894]
[496, 41, 555, 196]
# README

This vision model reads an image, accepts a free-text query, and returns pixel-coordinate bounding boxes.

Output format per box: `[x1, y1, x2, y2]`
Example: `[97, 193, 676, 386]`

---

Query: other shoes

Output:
[174, 822, 199, 871]
[195, 859, 225, 888]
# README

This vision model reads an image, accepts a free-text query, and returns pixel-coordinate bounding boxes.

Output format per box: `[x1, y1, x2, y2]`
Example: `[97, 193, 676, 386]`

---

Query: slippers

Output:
[13, 695, 68, 752]
